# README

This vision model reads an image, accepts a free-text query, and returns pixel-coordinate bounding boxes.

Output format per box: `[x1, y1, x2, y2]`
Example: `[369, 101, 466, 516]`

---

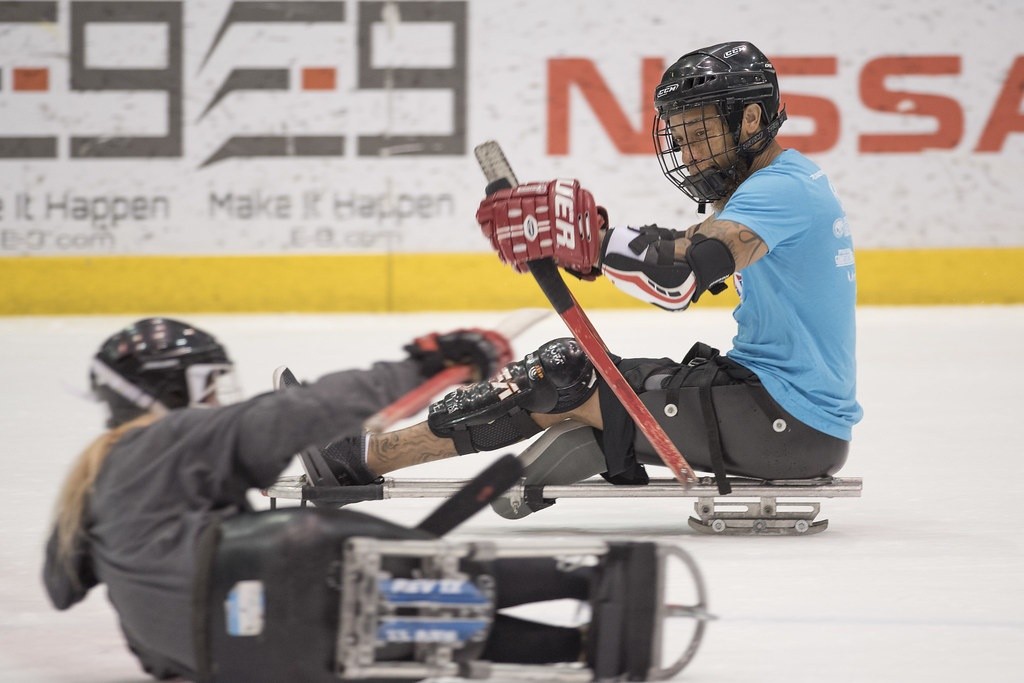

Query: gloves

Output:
[401, 327, 513, 384]
[475, 179, 608, 282]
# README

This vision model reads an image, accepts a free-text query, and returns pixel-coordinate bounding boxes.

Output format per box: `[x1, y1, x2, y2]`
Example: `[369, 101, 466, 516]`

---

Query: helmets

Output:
[654, 40, 780, 156]
[86, 317, 235, 430]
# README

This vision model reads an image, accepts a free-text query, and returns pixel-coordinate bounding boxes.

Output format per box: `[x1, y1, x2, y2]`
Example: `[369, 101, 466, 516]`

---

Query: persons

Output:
[274, 41, 865, 513]
[42, 319, 604, 682]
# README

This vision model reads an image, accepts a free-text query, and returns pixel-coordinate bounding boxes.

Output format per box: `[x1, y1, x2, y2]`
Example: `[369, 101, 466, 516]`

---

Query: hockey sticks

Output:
[483, 178, 700, 491]
[411, 452, 529, 540]
[363, 310, 553, 435]
[476, 140, 612, 359]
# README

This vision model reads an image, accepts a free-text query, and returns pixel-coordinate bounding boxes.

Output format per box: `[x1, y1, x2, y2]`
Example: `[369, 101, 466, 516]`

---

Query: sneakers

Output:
[273, 366, 385, 513]
